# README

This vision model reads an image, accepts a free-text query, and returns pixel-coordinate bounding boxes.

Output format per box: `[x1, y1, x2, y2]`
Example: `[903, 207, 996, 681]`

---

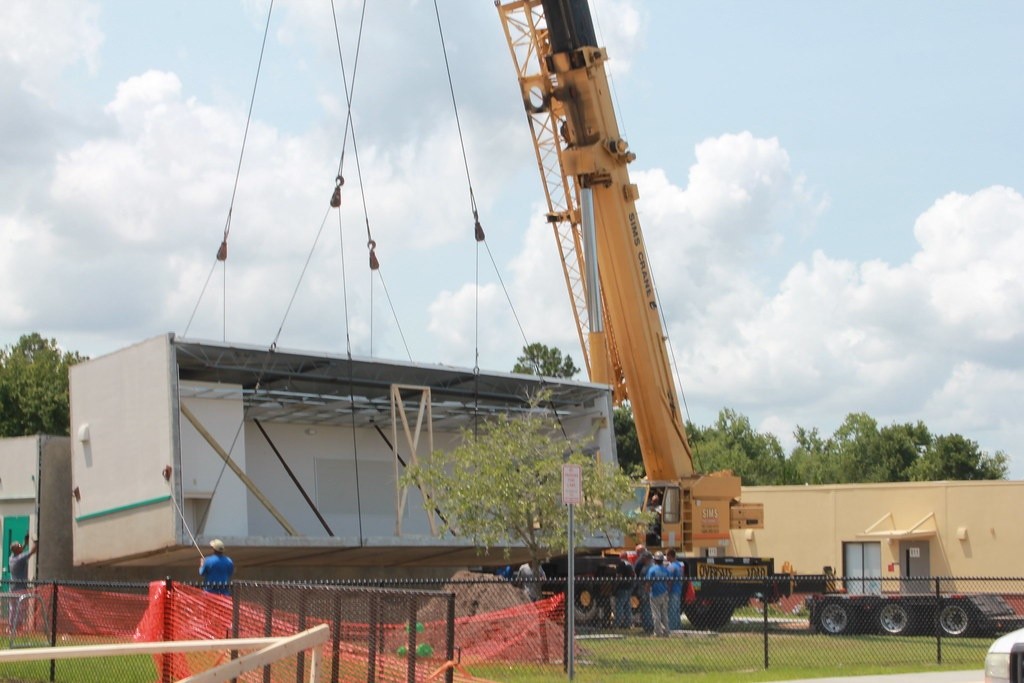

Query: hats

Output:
[654, 551, 664, 561]
[209, 539, 226, 552]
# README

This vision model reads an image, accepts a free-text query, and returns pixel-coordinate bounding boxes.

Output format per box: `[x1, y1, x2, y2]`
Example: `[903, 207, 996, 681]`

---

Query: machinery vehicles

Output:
[177, 3, 829, 637]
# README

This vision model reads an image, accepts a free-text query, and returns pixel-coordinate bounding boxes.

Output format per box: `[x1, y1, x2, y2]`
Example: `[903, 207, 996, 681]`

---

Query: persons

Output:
[6, 535, 38, 634]
[643, 494, 662, 545]
[666, 548, 684, 630]
[199, 539, 234, 597]
[612, 544, 654, 635]
[516, 562, 546, 602]
[646, 551, 671, 638]
